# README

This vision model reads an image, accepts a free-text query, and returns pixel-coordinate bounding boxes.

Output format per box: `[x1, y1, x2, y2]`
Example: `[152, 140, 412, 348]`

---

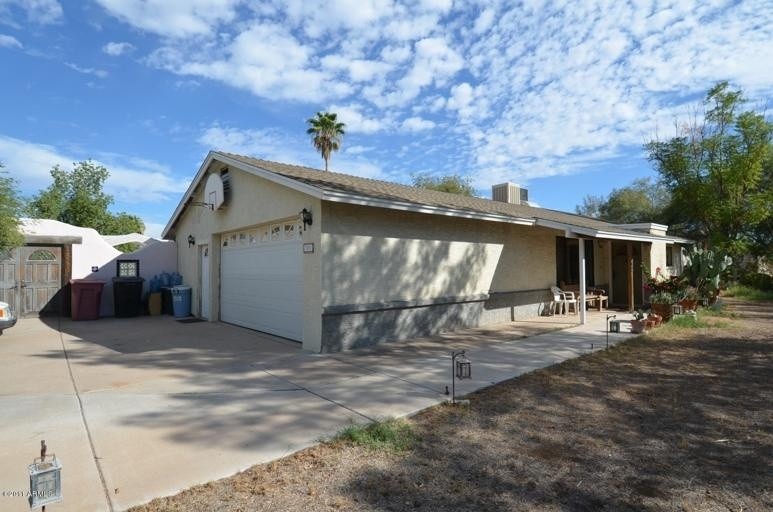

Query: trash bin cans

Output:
[161, 285, 191, 318]
[112, 276, 145, 318]
[69, 279, 107, 321]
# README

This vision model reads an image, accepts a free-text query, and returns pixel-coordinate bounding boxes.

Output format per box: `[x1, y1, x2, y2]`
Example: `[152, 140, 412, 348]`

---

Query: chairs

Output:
[550, 286, 577, 318]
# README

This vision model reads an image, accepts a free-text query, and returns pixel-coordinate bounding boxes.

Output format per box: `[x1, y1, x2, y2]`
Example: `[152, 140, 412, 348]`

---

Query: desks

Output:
[576, 294, 609, 312]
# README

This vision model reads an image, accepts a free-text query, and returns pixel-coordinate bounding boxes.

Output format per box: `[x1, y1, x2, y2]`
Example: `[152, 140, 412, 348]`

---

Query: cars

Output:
[0, 301, 15, 336]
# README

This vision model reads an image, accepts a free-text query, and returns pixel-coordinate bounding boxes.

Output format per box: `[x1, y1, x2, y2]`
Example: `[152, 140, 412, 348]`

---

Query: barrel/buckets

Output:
[150, 276, 161, 293]
[159, 270, 170, 285]
[172, 287, 191, 319]
[170, 270, 183, 285]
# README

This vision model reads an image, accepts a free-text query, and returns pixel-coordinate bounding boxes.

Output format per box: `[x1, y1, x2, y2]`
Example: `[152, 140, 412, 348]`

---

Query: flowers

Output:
[639, 260, 680, 293]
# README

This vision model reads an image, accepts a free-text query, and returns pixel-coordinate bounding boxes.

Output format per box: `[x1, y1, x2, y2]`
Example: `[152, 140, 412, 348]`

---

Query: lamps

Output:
[298, 207, 312, 231]
[186, 234, 195, 249]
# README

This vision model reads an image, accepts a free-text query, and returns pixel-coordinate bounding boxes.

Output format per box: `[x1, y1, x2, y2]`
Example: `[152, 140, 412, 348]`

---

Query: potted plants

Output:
[628, 284, 698, 333]
